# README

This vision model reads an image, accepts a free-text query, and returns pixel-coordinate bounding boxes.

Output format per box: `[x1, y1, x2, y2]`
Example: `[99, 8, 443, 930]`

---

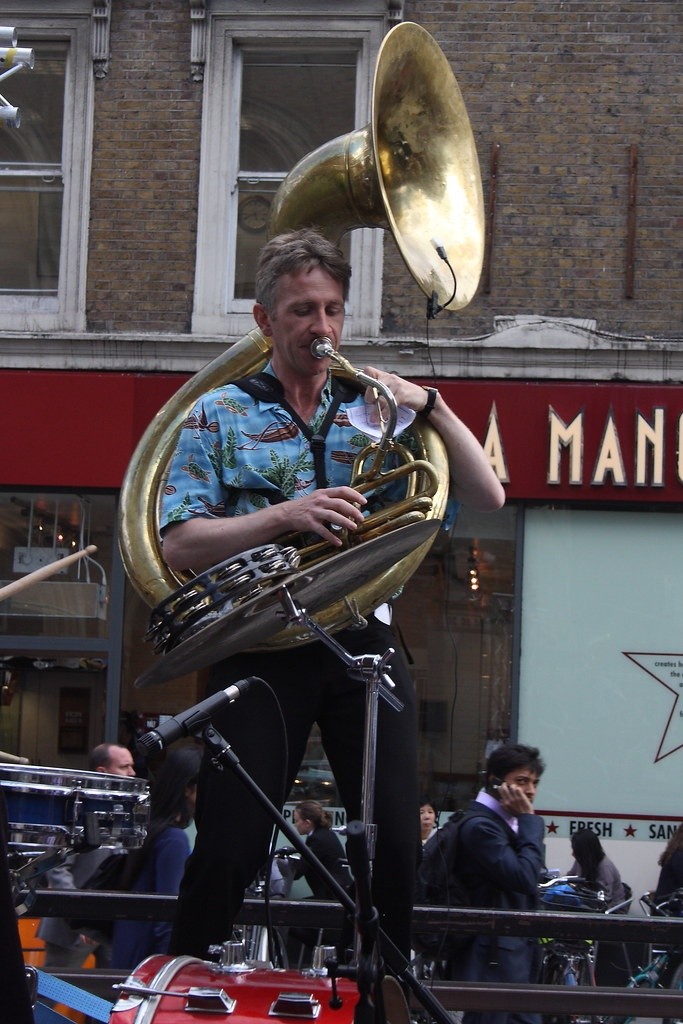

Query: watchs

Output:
[418, 385, 438, 417]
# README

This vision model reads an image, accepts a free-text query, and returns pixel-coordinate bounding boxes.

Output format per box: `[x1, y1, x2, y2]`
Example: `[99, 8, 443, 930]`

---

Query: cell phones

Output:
[485, 777, 510, 802]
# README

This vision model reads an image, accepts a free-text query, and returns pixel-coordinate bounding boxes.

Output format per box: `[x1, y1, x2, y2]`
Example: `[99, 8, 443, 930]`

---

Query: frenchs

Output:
[113, 22, 486, 657]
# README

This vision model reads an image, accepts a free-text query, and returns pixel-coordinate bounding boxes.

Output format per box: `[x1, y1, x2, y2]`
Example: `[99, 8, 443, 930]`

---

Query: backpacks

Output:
[414, 810, 508, 960]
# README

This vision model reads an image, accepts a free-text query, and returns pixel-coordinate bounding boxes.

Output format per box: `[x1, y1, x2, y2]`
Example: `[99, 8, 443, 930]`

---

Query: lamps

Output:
[35, 516, 78, 548]
[467, 546, 481, 592]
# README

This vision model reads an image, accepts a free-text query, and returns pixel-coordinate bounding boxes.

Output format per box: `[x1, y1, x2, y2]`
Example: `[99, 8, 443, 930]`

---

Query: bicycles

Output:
[538, 872, 683, 1024]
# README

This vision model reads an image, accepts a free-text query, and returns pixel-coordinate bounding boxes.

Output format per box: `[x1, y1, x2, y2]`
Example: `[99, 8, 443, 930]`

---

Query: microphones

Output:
[133, 676, 259, 760]
[427, 237, 457, 321]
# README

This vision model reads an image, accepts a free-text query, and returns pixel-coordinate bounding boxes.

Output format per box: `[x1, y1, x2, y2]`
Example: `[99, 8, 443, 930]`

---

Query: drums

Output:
[0, 763, 152, 850]
[110, 952, 361, 1024]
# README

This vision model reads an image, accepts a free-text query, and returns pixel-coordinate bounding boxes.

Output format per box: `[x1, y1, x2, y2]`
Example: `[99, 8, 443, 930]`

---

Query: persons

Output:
[96, 746, 195, 970]
[422, 745, 542, 1024]
[158, 228, 507, 973]
[0, 742, 683, 1024]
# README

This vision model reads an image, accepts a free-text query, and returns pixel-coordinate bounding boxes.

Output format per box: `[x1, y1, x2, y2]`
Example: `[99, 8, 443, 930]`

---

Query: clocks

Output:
[239, 195, 272, 234]
